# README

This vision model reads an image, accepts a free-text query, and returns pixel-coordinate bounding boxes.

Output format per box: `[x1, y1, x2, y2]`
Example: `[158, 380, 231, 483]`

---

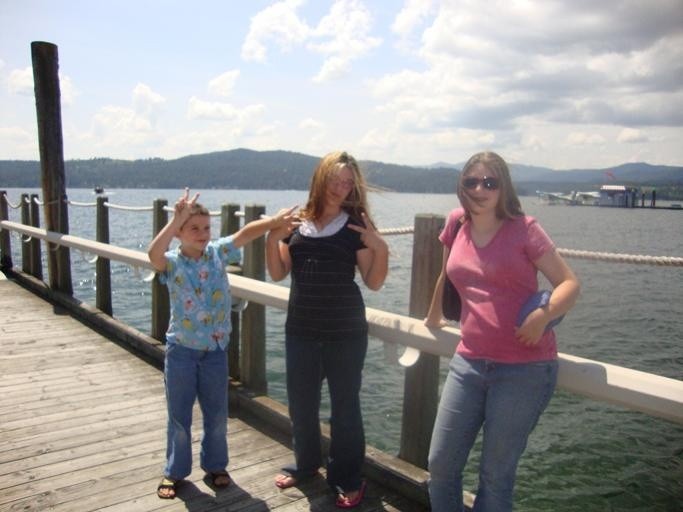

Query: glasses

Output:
[460, 175, 499, 193]
[328, 174, 355, 189]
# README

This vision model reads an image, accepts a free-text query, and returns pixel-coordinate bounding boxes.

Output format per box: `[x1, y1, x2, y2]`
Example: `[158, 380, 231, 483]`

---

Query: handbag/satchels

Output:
[440, 211, 464, 323]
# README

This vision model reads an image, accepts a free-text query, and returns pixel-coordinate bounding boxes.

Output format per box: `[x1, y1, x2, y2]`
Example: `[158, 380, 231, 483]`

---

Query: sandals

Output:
[275, 471, 319, 489]
[155, 476, 179, 500]
[209, 469, 230, 488]
[335, 480, 366, 507]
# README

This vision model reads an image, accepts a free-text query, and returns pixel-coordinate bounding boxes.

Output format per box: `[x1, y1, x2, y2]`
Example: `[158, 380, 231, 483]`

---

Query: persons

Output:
[424, 150, 580, 509]
[265, 153, 388, 507]
[146, 188, 300, 500]
